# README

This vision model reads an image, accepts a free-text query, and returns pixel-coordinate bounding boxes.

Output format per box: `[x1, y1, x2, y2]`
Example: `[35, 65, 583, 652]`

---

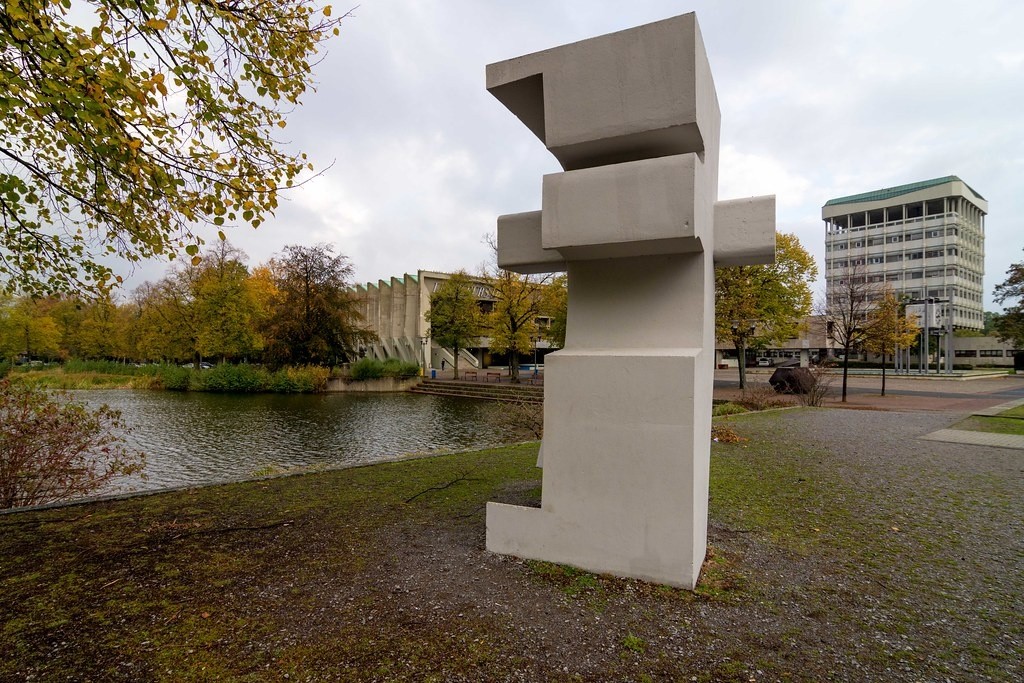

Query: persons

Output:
[441, 357, 446, 371]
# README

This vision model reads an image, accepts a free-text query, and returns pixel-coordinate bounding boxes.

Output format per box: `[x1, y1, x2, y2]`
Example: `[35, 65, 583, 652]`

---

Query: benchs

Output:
[525, 374, 544, 387]
[459, 371, 479, 382]
[481, 372, 501, 384]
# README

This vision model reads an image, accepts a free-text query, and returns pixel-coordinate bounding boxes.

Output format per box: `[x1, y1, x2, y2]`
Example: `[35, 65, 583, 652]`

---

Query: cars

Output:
[182, 362, 214, 369]
[759, 357, 775, 367]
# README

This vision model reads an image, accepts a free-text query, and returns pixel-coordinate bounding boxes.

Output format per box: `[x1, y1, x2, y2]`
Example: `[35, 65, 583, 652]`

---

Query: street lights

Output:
[530, 334, 542, 387]
[733, 322, 756, 389]
[420, 340, 428, 378]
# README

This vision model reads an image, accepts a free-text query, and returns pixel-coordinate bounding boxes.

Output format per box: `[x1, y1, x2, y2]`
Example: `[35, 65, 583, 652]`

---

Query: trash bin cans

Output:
[534, 370, 538, 375]
[432, 370, 436, 379]
